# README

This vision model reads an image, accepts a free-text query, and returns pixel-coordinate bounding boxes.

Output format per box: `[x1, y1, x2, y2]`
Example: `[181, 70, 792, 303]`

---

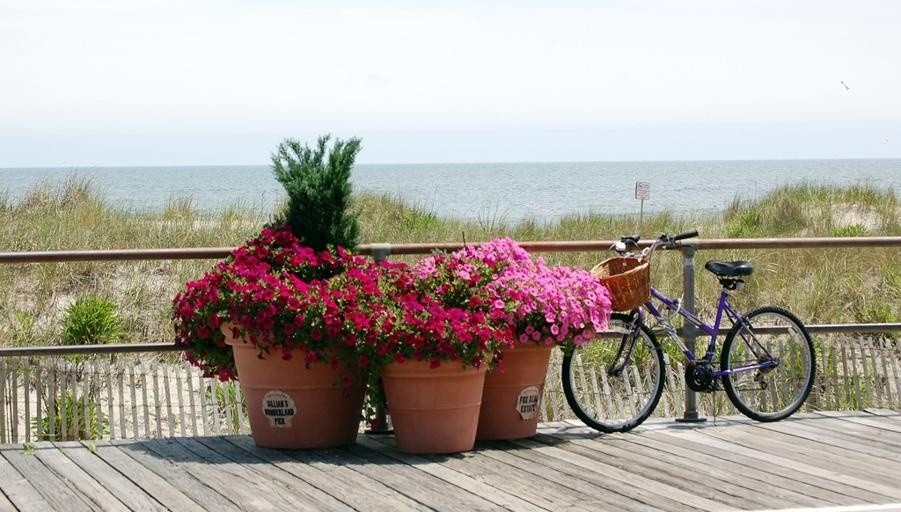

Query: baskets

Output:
[588, 254, 654, 312]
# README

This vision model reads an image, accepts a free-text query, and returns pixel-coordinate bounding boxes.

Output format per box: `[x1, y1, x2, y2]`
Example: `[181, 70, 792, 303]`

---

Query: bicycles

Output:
[560, 225, 819, 434]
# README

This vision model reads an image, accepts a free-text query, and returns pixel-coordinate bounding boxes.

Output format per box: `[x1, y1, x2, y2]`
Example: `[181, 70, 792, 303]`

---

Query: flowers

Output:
[157, 222, 614, 388]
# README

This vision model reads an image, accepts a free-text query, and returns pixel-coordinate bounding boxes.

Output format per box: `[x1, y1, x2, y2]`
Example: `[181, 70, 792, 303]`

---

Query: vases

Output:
[383, 340, 488, 456]
[477, 333, 555, 447]
[223, 320, 370, 456]
